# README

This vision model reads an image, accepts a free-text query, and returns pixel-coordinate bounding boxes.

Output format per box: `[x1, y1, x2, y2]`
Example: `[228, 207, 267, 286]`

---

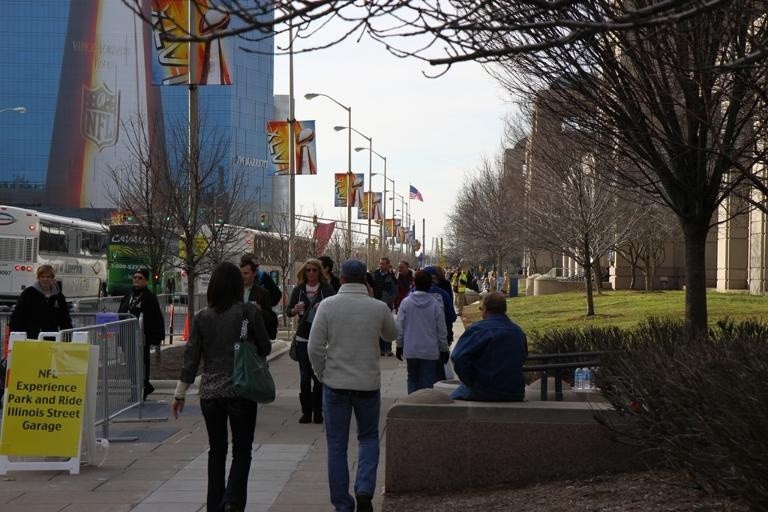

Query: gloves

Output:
[439, 348, 451, 365]
[396, 346, 404, 362]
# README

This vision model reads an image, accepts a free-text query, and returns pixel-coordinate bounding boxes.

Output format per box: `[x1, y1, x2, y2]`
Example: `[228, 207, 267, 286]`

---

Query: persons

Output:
[317, 256, 341, 296]
[406, 267, 457, 381]
[117, 269, 165, 402]
[372, 257, 397, 356]
[395, 260, 416, 361]
[396, 270, 449, 395]
[287, 259, 334, 422]
[467, 270, 479, 292]
[171, 261, 272, 512]
[450, 266, 468, 317]
[502, 273, 510, 298]
[238, 261, 269, 328]
[448, 292, 526, 402]
[307, 259, 400, 512]
[9, 265, 74, 342]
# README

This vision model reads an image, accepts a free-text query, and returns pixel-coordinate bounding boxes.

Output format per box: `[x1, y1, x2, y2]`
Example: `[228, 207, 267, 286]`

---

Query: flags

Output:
[410, 185, 423, 202]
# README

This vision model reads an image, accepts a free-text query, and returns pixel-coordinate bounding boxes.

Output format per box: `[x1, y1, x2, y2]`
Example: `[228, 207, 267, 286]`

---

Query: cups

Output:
[298, 301, 305, 316]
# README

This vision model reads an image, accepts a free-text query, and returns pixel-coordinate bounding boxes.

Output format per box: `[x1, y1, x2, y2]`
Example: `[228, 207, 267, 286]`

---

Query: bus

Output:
[96, 217, 182, 306]
[1, 204, 116, 319]
[171, 220, 316, 317]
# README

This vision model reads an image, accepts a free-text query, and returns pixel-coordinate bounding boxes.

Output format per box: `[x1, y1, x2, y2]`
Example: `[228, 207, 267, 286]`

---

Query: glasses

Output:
[479, 307, 482, 311]
[306, 267, 319, 272]
[133, 275, 145, 281]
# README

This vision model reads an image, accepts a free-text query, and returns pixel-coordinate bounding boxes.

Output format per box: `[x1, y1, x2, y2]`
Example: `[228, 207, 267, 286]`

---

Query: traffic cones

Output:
[179, 310, 189, 340]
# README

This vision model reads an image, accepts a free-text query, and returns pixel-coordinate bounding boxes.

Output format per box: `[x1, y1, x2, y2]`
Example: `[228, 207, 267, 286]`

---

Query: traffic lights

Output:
[260, 213, 269, 229]
[312, 213, 318, 228]
[216, 206, 224, 225]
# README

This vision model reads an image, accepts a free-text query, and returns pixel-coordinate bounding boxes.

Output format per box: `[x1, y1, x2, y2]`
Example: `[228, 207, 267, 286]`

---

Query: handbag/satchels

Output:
[289, 337, 299, 362]
[231, 301, 276, 405]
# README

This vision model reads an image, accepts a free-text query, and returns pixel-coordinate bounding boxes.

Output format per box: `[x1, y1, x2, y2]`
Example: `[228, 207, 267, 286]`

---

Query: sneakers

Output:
[380, 349, 394, 358]
[355, 491, 374, 511]
[128, 382, 156, 404]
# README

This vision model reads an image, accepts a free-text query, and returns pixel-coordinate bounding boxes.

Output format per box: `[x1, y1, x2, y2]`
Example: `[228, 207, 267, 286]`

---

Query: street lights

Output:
[353, 145, 388, 273]
[369, 170, 396, 269]
[332, 124, 375, 274]
[382, 187, 418, 273]
[0, 104, 28, 118]
[301, 89, 354, 265]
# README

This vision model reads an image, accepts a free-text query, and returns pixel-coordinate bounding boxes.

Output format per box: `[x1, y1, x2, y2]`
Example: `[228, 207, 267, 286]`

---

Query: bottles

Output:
[576, 366, 602, 391]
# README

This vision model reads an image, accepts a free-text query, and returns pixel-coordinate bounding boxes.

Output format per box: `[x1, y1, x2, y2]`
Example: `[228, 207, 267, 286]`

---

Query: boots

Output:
[312, 391, 325, 424]
[298, 392, 312, 423]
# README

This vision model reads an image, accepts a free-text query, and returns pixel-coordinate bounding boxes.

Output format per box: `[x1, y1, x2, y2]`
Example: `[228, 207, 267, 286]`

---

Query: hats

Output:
[134, 268, 150, 282]
[341, 261, 368, 280]
